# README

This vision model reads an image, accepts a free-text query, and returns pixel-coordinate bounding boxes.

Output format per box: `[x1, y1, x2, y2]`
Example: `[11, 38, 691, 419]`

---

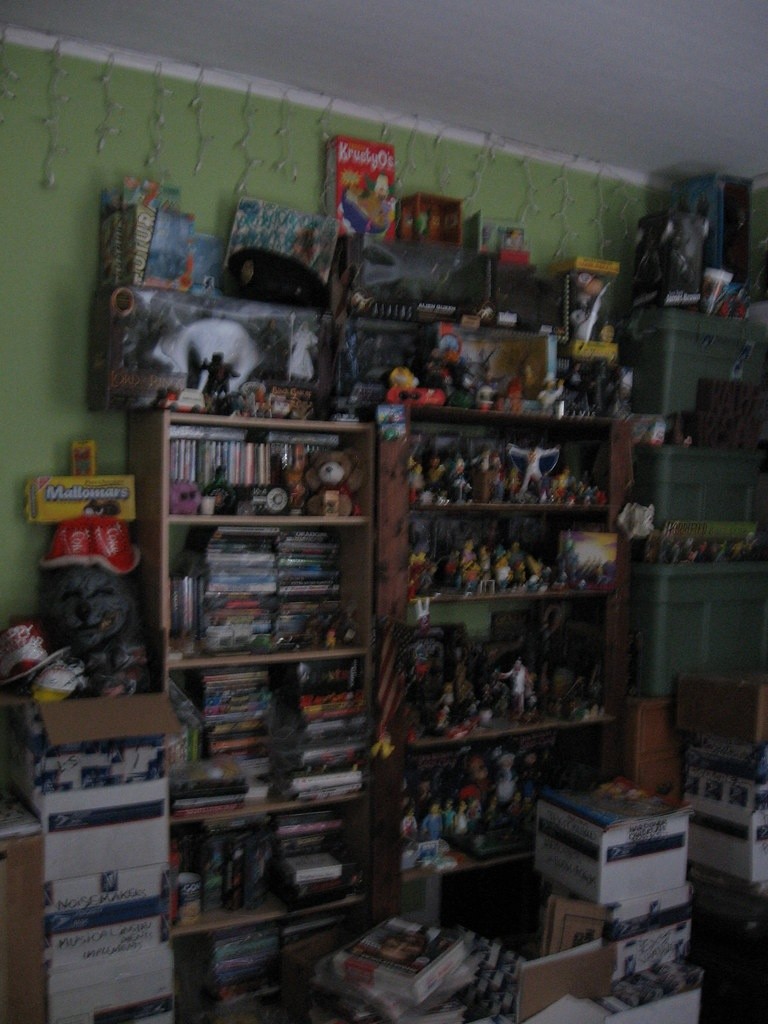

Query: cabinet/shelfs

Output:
[128, 409, 376, 1011]
[375, 399, 631, 928]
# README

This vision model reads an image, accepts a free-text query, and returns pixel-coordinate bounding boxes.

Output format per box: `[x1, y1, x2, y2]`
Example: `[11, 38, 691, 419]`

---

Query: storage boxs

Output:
[334, 738, 768, 1024]
[87, 183, 620, 413]
[0, 628, 176, 1024]
[325, 133, 398, 237]
[70, 441, 98, 475]
[22, 474, 136, 524]
[625, 309, 768, 881]
[280, 912, 345, 998]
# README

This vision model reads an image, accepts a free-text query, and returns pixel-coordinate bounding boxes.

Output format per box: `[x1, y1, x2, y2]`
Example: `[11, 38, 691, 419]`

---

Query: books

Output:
[167, 421, 489, 1024]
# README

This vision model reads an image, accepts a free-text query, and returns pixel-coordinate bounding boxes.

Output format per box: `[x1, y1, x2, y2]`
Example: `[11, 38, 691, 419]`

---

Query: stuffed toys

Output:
[36, 515, 147, 699]
[302, 448, 365, 515]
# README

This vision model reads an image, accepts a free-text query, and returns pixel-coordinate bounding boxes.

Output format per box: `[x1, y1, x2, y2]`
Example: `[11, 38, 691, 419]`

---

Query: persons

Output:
[399, 435, 604, 841]
[165, 352, 635, 425]
[645, 532, 761, 564]
